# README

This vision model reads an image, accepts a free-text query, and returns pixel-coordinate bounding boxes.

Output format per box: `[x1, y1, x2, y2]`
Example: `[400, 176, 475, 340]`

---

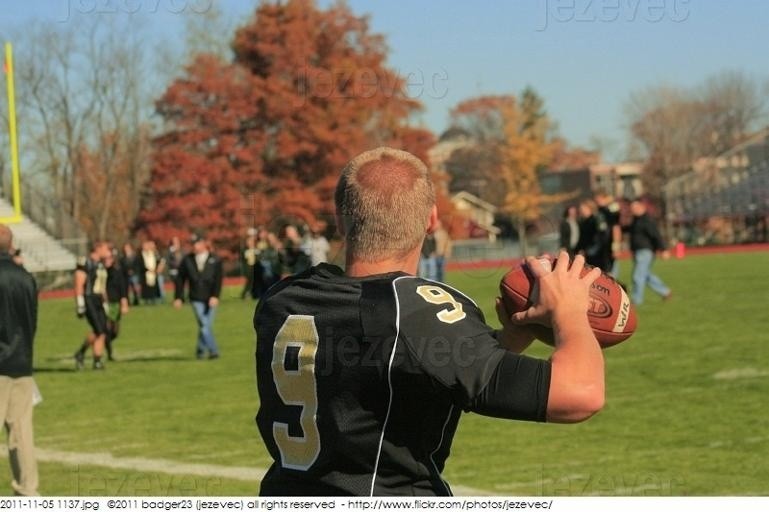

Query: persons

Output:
[72, 231, 186, 369]
[236, 220, 333, 302]
[174, 230, 223, 361]
[416, 228, 439, 283]
[252, 146, 606, 494]
[433, 216, 451, 283]
[0, 223, 41, 494]
[10, 247, 26, 269]
[559, 188, 675, 309]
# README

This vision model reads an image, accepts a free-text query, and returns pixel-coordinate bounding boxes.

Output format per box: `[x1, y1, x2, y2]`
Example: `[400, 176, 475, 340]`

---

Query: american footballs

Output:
[500, 258, 638, 349]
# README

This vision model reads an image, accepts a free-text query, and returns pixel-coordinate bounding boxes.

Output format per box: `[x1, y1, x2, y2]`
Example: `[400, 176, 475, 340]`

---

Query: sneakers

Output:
[75, 351, 114, 370]
[197, 354, 217, 360]
